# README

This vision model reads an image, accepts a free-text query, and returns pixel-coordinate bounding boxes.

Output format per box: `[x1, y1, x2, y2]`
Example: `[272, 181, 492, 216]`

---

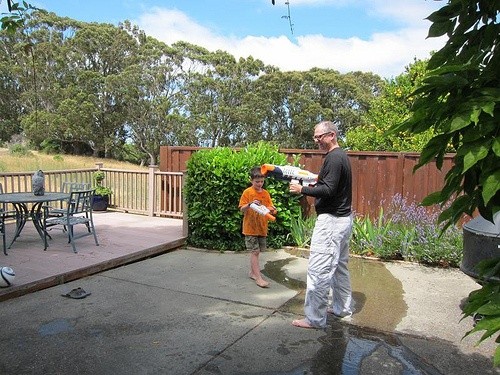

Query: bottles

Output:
[32, 170, 46, 196]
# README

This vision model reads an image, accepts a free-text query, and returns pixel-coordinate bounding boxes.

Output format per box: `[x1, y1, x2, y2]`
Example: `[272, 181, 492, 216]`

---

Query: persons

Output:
[289, 121, 353, 329]
[238, 167, 277, 287]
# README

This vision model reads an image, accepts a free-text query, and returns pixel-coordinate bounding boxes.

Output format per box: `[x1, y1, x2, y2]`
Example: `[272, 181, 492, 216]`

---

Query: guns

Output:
[248, 202, 275, 221]
[261, 163, 318, 192]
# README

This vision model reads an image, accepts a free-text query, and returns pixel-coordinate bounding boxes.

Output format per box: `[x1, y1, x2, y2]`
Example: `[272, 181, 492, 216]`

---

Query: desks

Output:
[1, 193, 70, 251]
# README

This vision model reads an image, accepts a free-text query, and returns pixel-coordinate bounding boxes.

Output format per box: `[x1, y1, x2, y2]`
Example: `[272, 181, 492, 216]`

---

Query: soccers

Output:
[0, 267, 15, 286]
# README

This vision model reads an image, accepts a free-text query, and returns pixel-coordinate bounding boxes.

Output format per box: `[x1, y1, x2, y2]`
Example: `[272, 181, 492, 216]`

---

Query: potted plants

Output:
[90, 172, 113, 211]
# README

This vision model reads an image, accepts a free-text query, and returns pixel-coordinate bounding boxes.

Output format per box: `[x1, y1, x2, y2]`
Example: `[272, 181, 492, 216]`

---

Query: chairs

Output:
[0, 182, 99, 256]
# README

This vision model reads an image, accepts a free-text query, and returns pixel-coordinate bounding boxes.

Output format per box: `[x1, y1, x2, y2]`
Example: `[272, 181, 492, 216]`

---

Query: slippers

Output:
[76, 287, 92, 296]
[61, 289, 87, 299]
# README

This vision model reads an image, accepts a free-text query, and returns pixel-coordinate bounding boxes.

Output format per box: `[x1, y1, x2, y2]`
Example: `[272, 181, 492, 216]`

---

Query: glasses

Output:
[312, 131, 332, 139]
